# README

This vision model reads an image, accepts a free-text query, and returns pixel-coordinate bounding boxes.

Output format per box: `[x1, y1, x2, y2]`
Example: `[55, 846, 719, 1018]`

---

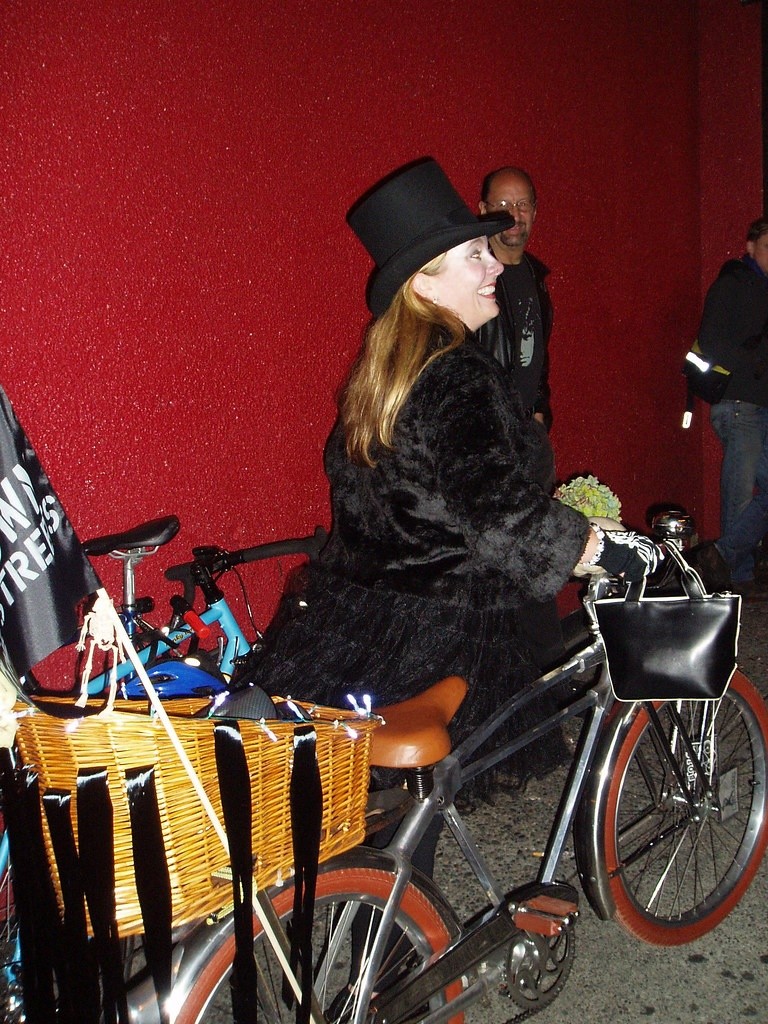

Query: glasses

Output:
[485, 198, 533, 214]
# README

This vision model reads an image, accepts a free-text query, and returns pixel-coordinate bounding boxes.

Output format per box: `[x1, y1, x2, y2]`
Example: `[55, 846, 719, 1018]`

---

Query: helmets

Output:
[117, 659, 226, 699]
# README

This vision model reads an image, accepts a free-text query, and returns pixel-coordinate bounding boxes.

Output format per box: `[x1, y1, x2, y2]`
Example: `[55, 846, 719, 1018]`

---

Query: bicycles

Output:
[0, 514, 334, 1023]
[164, 500, 768, 1024]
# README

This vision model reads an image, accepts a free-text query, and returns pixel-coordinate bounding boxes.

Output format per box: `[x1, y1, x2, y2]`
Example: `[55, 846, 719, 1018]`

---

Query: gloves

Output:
[579, 523, 664, 584]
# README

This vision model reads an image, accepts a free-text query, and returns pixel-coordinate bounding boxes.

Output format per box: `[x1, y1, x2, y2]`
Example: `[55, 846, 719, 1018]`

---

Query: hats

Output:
[345, 156, 513, 315]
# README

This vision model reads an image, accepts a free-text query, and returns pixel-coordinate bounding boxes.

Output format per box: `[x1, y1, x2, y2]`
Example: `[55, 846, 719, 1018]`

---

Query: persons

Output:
[680, 214, 768, 601]
[470, 164, 555, 496]
[238, 157, 663, 1024]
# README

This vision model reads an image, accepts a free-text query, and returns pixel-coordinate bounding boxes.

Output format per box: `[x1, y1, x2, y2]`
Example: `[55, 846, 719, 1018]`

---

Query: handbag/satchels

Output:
[681, 339, 730, 429]
[593, 540, 742, 703]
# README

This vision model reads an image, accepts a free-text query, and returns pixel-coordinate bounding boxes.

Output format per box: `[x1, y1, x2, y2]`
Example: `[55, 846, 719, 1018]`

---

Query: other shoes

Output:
[697, 542, 732, 595]
[323, 986, 430, 1024]
[729, 579, 768, 601]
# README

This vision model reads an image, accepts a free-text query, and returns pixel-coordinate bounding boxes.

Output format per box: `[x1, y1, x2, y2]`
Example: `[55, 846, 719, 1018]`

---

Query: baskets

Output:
[13, 696, 383, 942]
